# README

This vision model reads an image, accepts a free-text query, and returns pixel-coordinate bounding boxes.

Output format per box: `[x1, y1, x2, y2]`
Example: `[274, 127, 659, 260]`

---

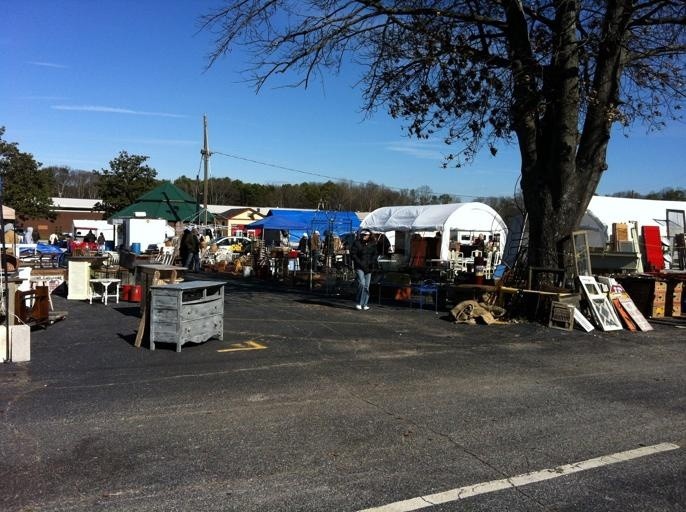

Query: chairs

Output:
[448, 250, 502, 280]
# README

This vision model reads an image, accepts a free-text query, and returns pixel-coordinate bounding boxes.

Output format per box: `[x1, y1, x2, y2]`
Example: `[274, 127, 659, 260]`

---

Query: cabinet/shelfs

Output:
[149, 280, 227, 352]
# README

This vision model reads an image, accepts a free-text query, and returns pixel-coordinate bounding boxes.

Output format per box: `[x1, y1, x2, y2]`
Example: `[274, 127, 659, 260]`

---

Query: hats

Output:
[361, 231, 370, 234]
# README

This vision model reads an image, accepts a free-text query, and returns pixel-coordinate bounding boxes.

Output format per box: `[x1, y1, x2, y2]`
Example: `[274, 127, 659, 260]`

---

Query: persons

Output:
[350, 229, 378, 311]
[1, 223, 527, 274]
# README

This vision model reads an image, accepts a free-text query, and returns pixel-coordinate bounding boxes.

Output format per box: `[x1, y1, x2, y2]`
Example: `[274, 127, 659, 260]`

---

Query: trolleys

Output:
[32, 278, 68, 326]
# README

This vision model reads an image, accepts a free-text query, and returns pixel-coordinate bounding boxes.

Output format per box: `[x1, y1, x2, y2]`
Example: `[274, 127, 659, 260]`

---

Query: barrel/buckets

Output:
[253, 263, 268, 282]
[242, 265, 251, 277]
[129, 284, 142, 303]
[120, 284, 131, 301]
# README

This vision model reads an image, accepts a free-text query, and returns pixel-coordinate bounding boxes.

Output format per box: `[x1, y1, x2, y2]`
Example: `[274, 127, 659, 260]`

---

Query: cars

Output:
[205, 237, 322, 276]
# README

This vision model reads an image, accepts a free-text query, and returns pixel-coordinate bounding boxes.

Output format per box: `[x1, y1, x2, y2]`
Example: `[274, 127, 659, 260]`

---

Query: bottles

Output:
[73, 242, 90, 257]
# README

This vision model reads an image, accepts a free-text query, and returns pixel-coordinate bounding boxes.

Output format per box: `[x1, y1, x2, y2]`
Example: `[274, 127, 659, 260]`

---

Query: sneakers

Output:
[356, 304, 362, 310]
[363, 306, 370, 311]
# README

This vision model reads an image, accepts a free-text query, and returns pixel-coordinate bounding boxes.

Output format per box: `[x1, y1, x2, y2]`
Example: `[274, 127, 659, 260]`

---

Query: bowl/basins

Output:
[0, 254, 19, 273]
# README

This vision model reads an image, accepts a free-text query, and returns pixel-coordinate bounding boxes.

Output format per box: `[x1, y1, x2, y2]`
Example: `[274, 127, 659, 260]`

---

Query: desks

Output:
[88, 278, 121, 307]
